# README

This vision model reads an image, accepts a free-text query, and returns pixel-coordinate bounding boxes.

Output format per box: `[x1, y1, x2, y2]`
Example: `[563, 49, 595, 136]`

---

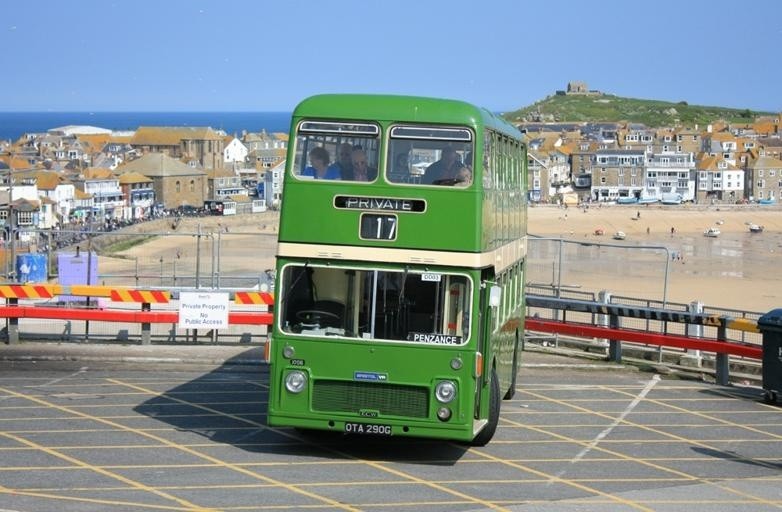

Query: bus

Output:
[268, 93, 527, 444]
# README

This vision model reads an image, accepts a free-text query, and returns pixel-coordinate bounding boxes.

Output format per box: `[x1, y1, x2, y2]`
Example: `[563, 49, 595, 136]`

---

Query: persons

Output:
[555, 193, 608, 221]
[41, 204, 222, 253]
[301, 142, 472, 188]
[647, 227, 649, 234]
[637, 211, 640, 218]
[671, 227, 674, 234]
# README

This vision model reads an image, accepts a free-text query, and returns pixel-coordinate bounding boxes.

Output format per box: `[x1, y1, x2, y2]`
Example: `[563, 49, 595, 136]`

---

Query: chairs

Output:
[312, 299, 344, 329]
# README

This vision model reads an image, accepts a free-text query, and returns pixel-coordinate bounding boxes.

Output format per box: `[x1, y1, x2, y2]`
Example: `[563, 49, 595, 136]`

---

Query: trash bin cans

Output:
[758, 309, 778, 401]
